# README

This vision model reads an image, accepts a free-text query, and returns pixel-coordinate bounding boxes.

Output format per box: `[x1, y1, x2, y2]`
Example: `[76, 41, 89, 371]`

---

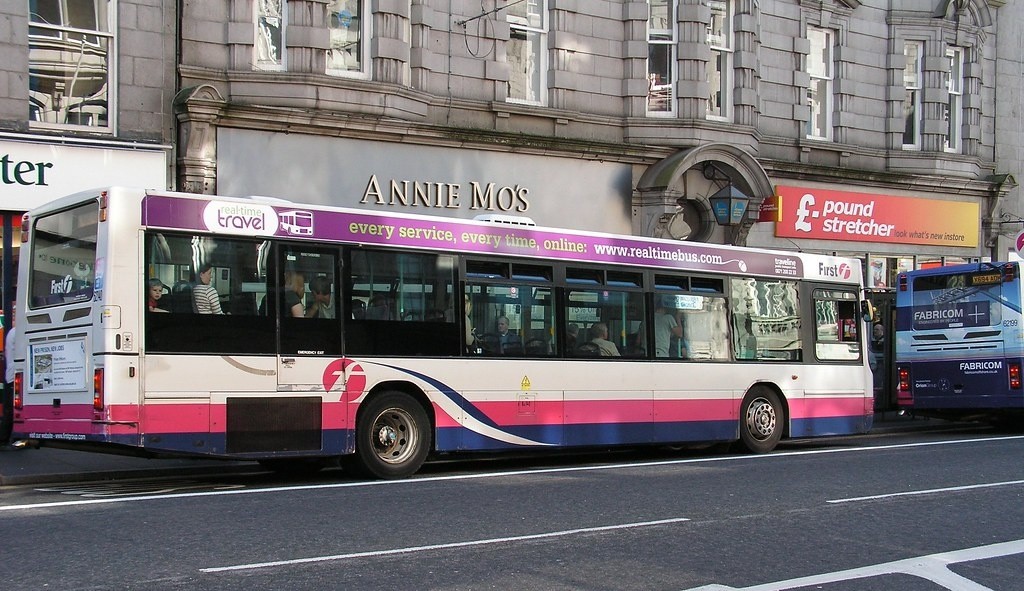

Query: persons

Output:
[493, 315, 521, 355]
[871, 324, 885, 353]
[148, 278, 171, 313]
[637, 299, 687, 360]
[0, 326, 19, 451]
[440, 293, 476, 354]
[566, 323, 621, 357]
[183, 262, 227, 315]
[304, 276, 356, 320]
[259, 271, 305, 319]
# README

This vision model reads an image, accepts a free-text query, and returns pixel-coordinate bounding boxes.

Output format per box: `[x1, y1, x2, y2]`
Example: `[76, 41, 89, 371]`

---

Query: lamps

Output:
[703, 162, 749, 226]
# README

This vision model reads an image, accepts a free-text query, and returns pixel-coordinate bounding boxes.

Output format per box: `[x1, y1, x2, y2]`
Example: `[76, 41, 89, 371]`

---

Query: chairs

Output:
[162, 282, 448, 326]
[464, 333, 646, 360]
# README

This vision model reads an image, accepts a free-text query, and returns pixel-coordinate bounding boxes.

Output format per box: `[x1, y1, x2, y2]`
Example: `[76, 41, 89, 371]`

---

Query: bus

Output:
[8, 186, 880, 482]
[890, 260, 1024, 428]
